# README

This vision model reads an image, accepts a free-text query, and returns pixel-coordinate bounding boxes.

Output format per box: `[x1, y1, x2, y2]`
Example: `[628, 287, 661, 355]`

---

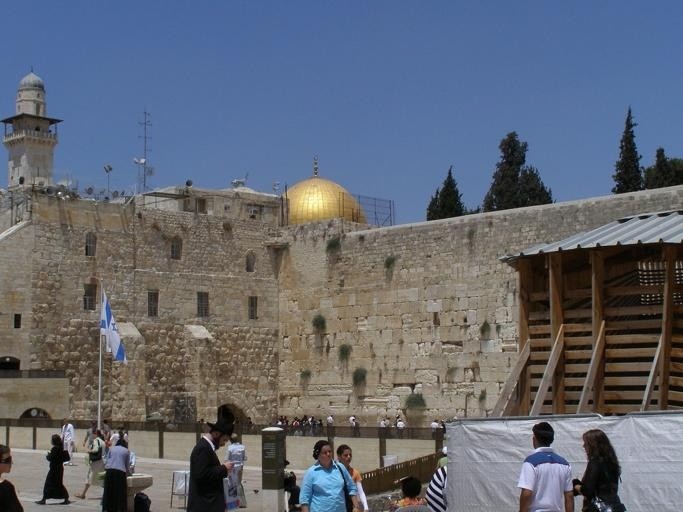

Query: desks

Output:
[170, 471, 191, 509]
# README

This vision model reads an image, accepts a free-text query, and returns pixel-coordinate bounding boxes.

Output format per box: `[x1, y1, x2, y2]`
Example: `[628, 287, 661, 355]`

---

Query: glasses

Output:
[1, 456, 12, 464]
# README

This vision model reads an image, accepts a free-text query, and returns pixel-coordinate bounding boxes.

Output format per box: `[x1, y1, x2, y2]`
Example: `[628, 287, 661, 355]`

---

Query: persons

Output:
[515, 420, 574, 512]
[247, 417, 252, 426]
[187, 418, 247, 511]
[0, 443, 25, 510]
[76, 420, 135, 511]
[573, 428, 627, 512]
[393, 447, 449, 511]
[430, 419, 447, 440]
[380, 414, 405, 438]
[327, 415, 335, 424]
[283, 440, 368, 510]
[349, 415, 356, 427]
[276, 414, 323, 436]
[35, 434, 69, 505]
[60, 418, 75, 466]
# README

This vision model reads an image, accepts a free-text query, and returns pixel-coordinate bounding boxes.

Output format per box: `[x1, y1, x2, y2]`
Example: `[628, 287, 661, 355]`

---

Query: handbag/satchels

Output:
[587, 492, 626, 512]
[88, 447, 102, 461]
[341, 485, 353, 512]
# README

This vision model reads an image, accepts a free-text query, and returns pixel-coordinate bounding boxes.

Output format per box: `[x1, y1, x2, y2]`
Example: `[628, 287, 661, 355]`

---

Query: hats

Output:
[206, 419, 235, 437]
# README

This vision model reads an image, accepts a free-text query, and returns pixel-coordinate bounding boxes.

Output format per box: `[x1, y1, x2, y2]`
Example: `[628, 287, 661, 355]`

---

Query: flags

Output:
[98, 289, 128, 367]
